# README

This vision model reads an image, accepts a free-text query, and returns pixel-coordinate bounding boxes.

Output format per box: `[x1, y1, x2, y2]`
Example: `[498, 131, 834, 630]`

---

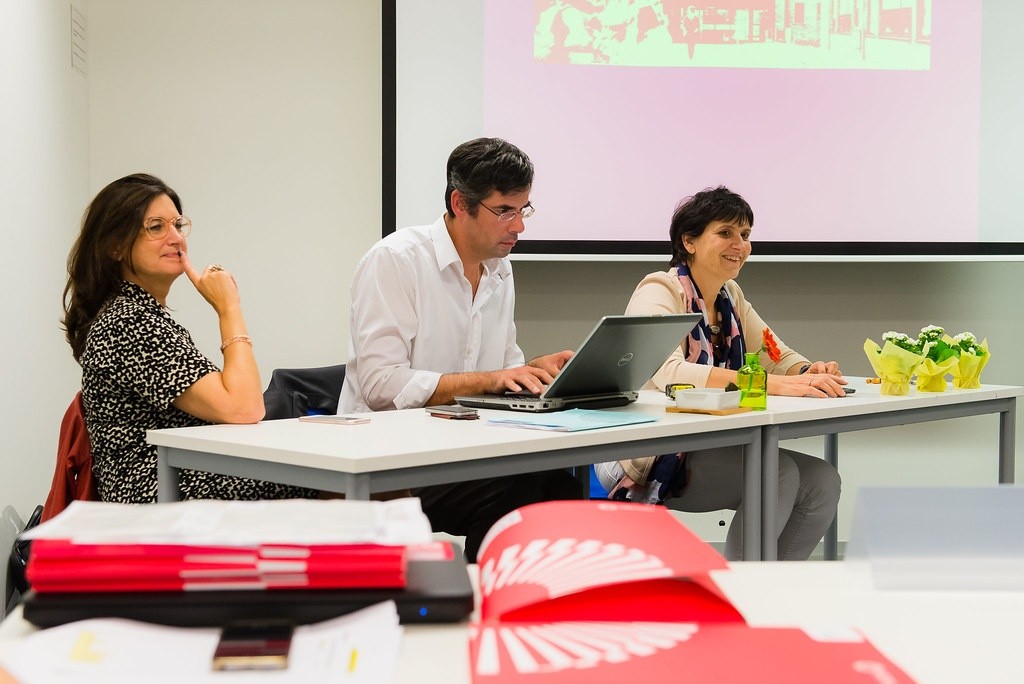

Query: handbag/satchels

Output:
[6, 505, 44, 616]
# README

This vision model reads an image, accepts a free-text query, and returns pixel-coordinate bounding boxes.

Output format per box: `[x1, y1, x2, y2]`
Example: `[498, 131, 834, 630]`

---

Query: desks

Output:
[145, 384, 1024, 562]
[0, 561, 1024, 684]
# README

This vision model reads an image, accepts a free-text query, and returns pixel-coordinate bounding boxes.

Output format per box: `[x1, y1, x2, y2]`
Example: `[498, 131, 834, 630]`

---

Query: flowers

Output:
[913, 325, 963, 393]
[744, 328, 780, 394]
[950, 331, 991, 390]
[877, 330, 922, 396]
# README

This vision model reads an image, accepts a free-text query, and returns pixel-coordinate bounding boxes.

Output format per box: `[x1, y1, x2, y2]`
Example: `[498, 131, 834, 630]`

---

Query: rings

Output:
[208, 264, 224, 272]
[808, 380, 813, 386]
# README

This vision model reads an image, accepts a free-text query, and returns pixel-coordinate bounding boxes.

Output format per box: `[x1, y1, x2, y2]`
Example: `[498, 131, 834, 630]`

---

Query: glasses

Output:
[471, 198, 535, 222]
[139, 215, 191, 240]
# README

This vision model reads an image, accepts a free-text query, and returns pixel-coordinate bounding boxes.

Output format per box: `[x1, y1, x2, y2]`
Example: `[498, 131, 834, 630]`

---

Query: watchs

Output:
[799, 364, 811, 375]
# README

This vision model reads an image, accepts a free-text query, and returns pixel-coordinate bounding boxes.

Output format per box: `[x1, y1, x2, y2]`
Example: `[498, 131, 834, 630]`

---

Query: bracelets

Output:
[220, 336, 253, 354]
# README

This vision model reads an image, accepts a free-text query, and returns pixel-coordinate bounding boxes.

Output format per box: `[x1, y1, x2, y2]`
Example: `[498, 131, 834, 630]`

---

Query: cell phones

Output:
[210, 621, 295, 671]
[299, 414, 371, 425]
[426, 406, 478, 416]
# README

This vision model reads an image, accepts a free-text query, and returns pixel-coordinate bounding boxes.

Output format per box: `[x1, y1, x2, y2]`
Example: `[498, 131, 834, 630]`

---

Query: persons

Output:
[336, 137, 575, 564]
[62, 173, 320, 504]
[594, 184, 848, 562]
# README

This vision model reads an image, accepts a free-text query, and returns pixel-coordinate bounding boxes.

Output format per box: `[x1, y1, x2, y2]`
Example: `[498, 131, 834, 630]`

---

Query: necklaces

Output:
[710, 325, 720, 335]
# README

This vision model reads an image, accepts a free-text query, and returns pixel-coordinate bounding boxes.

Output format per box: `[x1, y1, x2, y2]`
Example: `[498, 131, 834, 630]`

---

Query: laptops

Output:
[457, 312, 704, 413]
[22, 542, 475, 631]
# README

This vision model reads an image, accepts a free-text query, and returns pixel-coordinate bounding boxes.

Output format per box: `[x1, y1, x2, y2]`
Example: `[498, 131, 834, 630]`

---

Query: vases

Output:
[738, 355, 768, 412]
